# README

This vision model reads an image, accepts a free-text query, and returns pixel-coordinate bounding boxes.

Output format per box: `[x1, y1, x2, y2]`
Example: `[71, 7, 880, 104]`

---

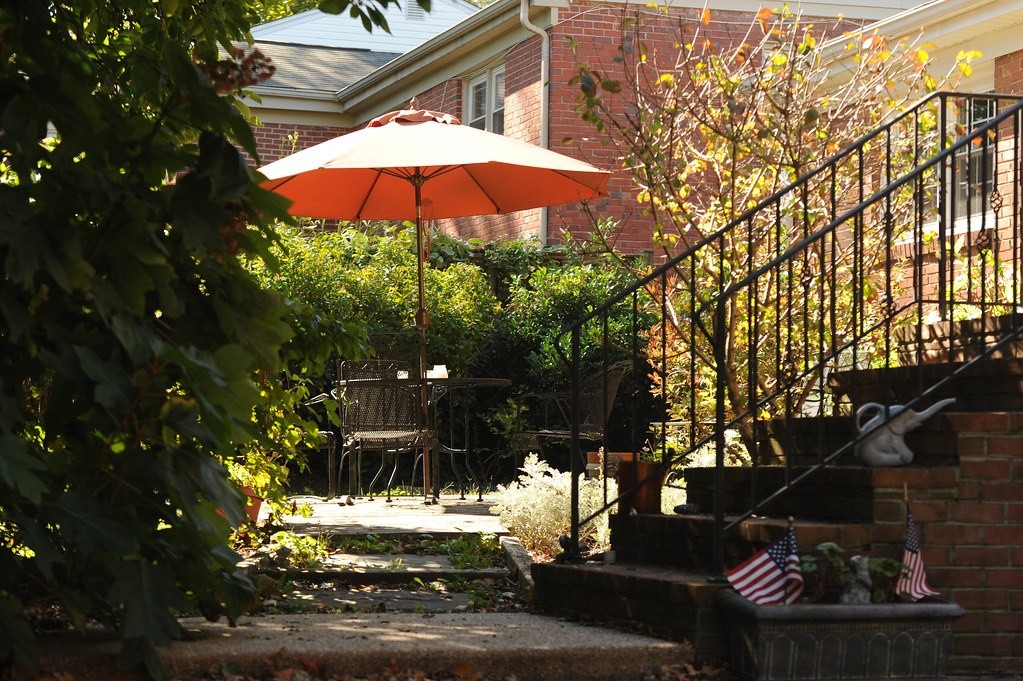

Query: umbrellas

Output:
[251, 99, 613, 495]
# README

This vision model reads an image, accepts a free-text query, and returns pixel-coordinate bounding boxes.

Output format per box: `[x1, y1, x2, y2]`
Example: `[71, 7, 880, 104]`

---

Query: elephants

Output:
[855, 398, 957, 464]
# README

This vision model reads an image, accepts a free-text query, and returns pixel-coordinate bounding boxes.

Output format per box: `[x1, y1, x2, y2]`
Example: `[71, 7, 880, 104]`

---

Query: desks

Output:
[337, 377, 513, 505]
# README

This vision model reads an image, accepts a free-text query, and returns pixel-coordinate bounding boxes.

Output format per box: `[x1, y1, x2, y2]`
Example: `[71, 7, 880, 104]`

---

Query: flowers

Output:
[638, 448, 655, 463]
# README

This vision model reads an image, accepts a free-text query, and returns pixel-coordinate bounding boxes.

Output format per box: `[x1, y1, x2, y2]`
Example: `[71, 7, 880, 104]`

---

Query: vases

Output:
[614, 460, 670, 515]
[215, 486, 264, 529]
[585, 452, 639, 480]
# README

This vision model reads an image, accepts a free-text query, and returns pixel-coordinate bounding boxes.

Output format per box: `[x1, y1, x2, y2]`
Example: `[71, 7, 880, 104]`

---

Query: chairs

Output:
[503, 359, 633, 475]
[334, 358, 425, 498]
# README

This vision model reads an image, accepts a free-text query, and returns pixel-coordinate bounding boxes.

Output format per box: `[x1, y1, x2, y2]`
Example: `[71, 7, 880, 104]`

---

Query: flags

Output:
[894, 505, 941, 601]
[725, 531, 804, 607]
